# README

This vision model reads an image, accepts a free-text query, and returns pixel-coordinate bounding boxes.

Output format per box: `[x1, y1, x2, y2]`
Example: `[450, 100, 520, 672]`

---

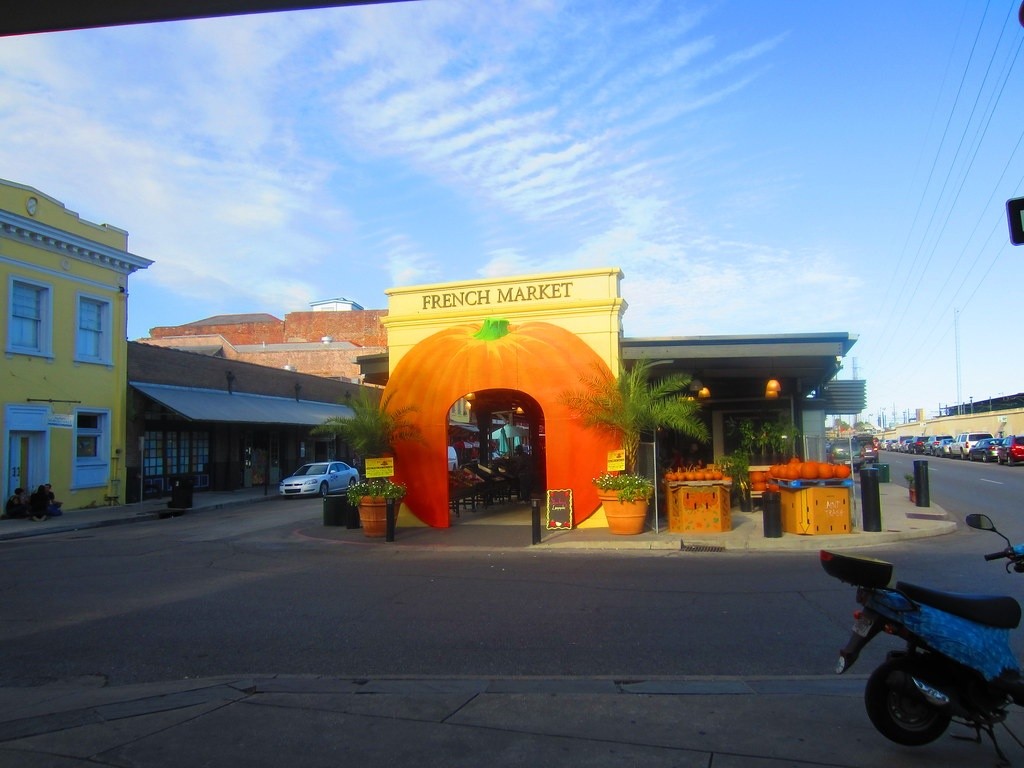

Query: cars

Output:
[969, 438, 1005, 462]
[997, 435, 1024, 466]
[936, 438, 954, 458]
[873, 435, 929, 455]
[279, 461, 359, 497]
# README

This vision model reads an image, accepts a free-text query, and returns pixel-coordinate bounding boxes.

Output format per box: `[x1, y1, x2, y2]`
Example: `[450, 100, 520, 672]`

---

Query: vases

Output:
[357, 495, 403, 538]
[596, 489, 651, 535]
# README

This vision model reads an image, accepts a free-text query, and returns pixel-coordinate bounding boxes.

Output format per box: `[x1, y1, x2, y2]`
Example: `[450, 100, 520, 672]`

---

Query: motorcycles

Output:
[818, 513, 1024, 767]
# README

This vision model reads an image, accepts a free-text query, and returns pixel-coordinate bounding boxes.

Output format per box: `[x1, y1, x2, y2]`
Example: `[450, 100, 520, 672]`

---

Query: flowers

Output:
[346, 476, 407, 506]
[592, 473, 655, 506]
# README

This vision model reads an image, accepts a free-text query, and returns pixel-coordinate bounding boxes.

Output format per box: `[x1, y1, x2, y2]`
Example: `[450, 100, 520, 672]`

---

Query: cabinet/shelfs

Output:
[449, 461, 519, 517]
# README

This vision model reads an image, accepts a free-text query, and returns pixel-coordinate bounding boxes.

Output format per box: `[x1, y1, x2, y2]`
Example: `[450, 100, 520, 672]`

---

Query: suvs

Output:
[924, 434, 954, 456]
[829, 437, 866, 471]
[853, 434, 878, 462]
[949, 432, 994, 460]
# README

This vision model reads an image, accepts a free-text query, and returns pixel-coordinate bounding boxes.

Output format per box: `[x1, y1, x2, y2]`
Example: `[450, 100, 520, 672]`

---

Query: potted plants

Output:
[714, 417, 800, 513]
[904, 473, 916, 503]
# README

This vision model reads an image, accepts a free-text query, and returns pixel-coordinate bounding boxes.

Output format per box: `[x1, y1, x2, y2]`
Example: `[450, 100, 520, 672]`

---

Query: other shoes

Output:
[32, 516, 39, 522]
[39, 514, 46, 522]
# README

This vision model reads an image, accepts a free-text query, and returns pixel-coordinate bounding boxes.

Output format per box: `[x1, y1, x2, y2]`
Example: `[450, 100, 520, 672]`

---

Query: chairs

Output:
[985, 442, 990, 444]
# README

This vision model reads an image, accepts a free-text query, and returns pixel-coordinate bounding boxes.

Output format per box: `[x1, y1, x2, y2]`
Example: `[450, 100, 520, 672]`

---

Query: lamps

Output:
[689, 379, 704, 391]
[765, 376, 782, 399]
[698, 386, 711, 398]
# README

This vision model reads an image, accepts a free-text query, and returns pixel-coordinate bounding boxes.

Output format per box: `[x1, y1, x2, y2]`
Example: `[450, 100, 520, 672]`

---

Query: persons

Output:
[668, 452, 684, 468]
[514, 444, 531, 502]
[7, 483, 63, 524]
[686, 443, 702, 467]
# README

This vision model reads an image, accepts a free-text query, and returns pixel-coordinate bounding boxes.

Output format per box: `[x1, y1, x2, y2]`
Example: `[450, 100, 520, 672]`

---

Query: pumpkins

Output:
[750, 456, 851, 492]
[663, 463, 723, 481]
